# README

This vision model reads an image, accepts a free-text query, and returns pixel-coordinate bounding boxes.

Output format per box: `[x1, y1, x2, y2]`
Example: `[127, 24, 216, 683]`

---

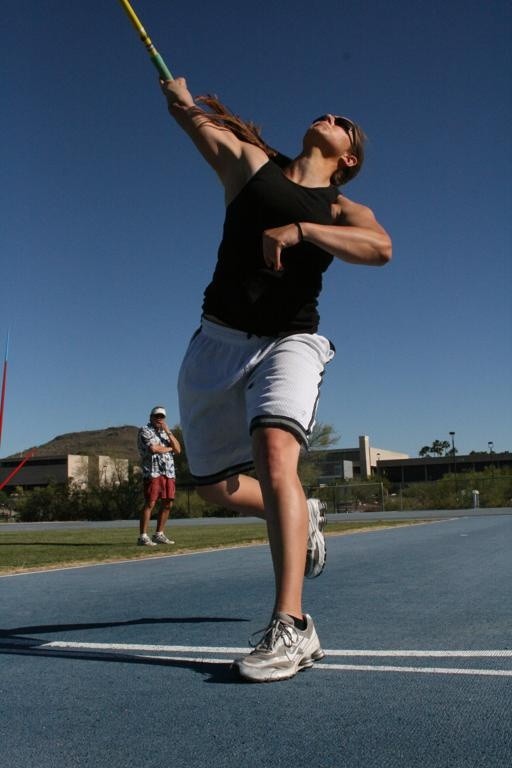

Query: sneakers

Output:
[305, 499, 326, 579]
[137, 537, 157, 546]
[229, 612, 324, 681]
[152, 535, 175, 544]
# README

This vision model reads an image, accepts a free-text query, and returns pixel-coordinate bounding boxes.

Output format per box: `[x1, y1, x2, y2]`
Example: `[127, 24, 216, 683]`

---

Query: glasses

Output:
[312, 117, 357, 151]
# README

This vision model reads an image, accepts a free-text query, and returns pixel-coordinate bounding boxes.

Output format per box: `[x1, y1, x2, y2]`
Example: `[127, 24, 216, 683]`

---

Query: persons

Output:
[159, 77, 394, 680]
[135, 405, 181, 546]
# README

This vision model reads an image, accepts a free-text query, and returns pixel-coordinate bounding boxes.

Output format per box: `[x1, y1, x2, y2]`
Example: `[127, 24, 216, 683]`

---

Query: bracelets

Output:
[167, 431, 172, 436]
[292, 218, 306, 245]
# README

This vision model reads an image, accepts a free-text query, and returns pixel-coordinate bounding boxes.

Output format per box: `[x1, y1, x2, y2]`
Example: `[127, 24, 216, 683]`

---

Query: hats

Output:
[151, 408, 166, 417]
